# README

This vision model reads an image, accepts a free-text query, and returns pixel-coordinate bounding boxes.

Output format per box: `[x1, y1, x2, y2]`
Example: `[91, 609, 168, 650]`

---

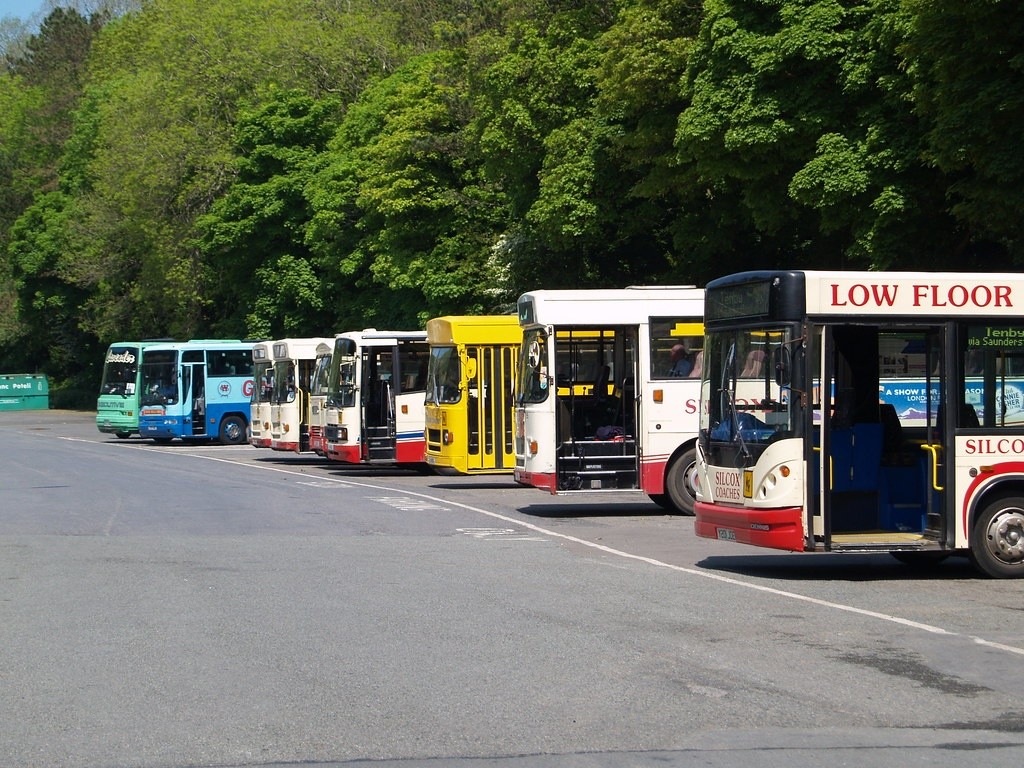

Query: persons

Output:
[667, 344, 693, 378]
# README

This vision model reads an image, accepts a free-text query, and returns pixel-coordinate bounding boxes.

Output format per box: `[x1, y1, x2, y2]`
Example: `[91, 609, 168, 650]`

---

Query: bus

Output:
[138, 342, 314, 446]
[424, 315, 788, 476]
[247, 329, 490, 477]
[694, 269, 1024, 580]
[96, 339, 242, 439]
[512, 286, 1024, 516]
